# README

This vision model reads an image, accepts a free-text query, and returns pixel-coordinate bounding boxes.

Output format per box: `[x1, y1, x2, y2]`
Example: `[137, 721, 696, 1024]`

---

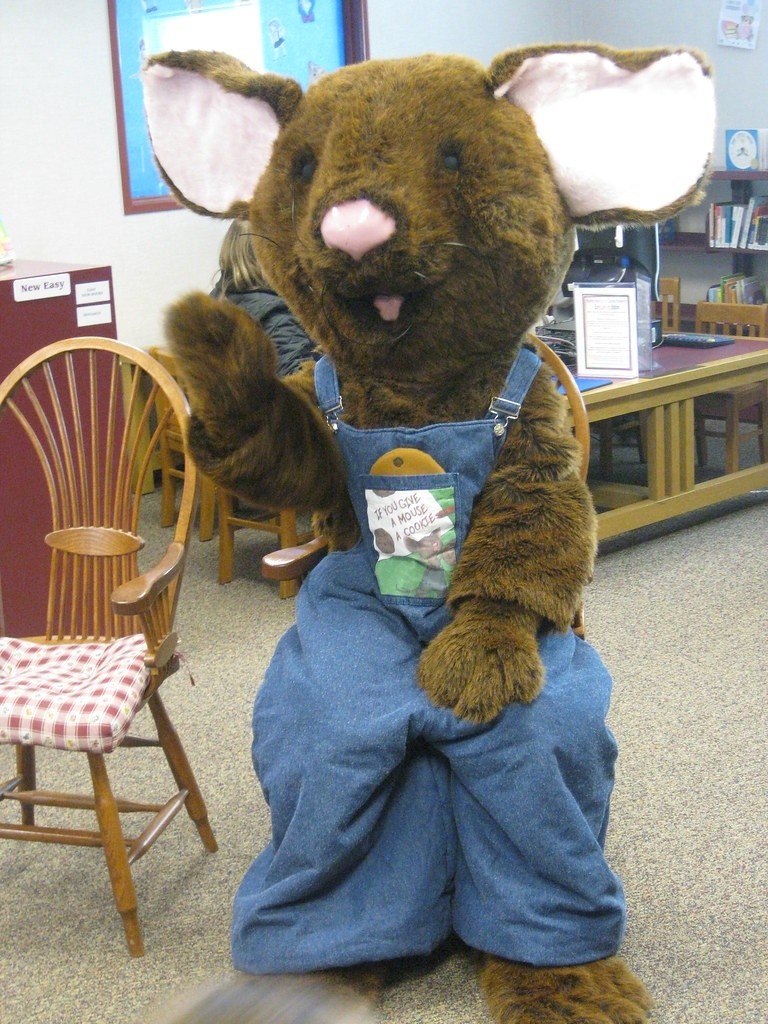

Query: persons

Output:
[202, 210, 321, 381]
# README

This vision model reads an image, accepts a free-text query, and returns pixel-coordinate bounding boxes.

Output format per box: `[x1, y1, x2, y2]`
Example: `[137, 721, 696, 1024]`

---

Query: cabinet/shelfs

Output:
[653, 171, 768, 325]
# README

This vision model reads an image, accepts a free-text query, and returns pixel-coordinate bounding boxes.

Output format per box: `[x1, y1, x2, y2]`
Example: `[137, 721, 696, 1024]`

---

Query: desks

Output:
[555, 331, 768, 541]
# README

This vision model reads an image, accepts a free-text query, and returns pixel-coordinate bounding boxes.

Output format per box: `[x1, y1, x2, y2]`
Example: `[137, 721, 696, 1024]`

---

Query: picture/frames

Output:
[106, 0, 371, 214]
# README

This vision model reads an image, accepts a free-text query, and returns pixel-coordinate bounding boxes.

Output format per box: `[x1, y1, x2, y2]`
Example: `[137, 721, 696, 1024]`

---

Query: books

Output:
[547, 375, 613, 397]
[705, 195, 768, 252]
[661, 331, 734, 350]
[706, 273, 765, 326]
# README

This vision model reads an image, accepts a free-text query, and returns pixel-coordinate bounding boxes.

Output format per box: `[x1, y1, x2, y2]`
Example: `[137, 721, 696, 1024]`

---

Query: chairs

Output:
[694, 301, 768, 475]
[0, 337, 220, 958]
[150, 346, 218, 541]
[599, 277, 681, 480]
[221, 487, 332, 600]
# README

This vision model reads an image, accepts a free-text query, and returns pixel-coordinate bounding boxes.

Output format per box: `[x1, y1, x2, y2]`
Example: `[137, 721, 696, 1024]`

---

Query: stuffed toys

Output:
[138, 43, 718, 1023]
